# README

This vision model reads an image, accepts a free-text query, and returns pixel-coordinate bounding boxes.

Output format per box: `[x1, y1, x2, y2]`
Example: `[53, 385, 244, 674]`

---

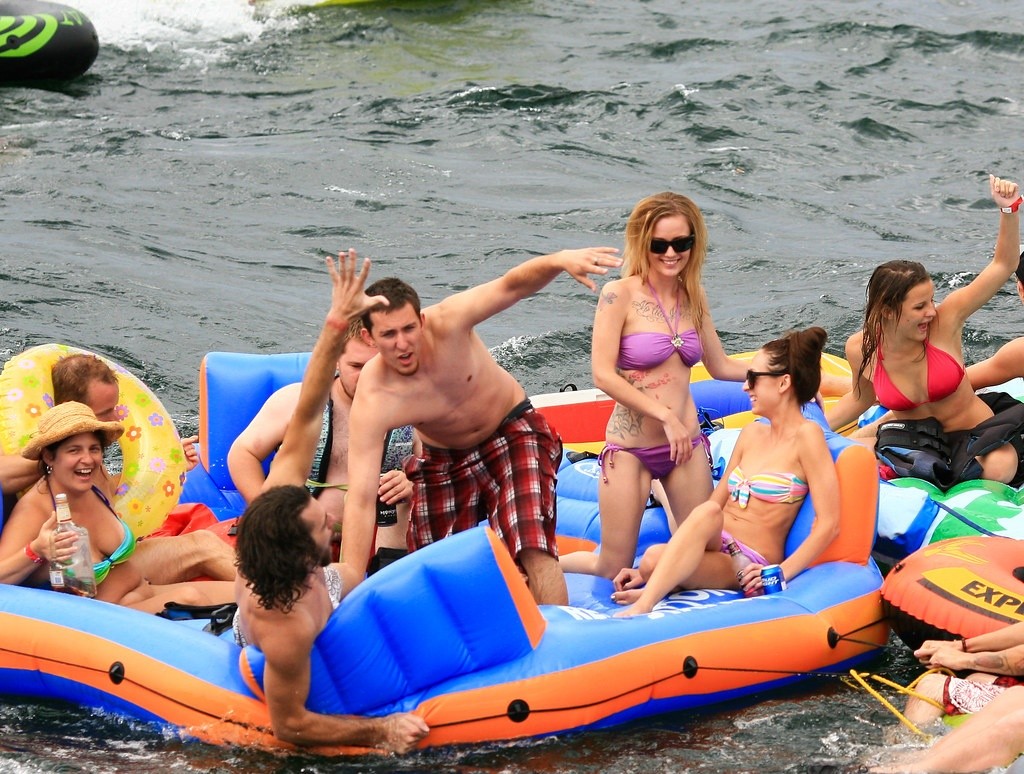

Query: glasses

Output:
[650, 234, 696, 255]
[747, 370, 786, 390]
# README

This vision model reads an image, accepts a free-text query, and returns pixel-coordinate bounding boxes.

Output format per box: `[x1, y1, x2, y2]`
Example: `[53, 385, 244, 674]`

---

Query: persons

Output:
[610, 326, 839, 617]
[559, 191, 753, 581]
[0, 354, 238, 612]
[824, 172, 1024, 486]
[226, 247, 623, 752]
[866, 567, 1024, 774]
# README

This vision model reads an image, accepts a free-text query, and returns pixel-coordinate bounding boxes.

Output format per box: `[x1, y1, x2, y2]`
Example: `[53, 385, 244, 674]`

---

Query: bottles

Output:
[52, 495, 97, 600]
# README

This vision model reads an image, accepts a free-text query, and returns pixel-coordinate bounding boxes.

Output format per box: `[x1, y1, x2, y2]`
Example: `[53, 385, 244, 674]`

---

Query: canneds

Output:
[760, 564, 787, 595]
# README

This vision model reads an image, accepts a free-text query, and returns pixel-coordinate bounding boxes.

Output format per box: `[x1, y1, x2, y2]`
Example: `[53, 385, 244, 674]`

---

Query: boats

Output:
[878, 479, 1024, 700]
[0, 484, 887, 763]
[186, 348, 866, 510]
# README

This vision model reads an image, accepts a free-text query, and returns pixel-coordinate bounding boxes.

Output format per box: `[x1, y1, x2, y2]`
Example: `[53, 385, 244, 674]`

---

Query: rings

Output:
[594, 256, 600, 266]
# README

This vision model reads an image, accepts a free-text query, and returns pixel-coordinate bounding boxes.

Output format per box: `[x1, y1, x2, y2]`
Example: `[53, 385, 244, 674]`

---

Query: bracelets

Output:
[1000, 194, 1024, 213]
[962, 637, 967, 653]
[26, 542, 46, 565]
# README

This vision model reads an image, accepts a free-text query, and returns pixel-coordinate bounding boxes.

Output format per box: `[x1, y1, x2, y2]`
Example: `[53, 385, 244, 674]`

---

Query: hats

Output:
[21, 400, 126, 461]
